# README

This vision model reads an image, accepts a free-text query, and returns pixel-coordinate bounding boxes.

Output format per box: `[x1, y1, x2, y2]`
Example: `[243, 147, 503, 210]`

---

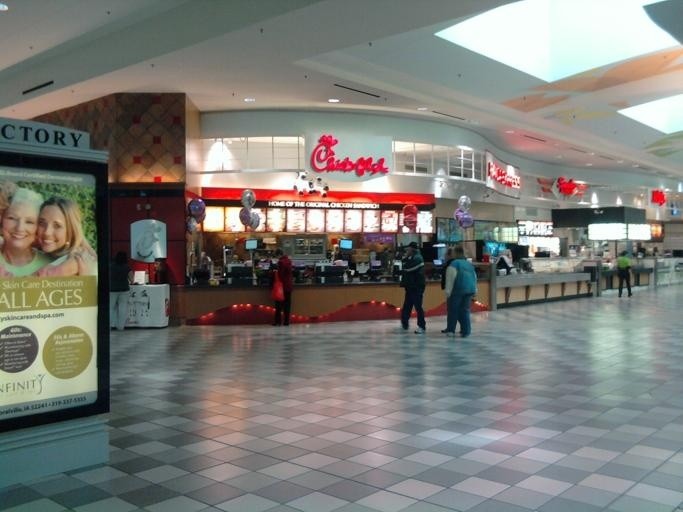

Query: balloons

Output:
[454, 195, 473, 228]
[403, 205, 417, 228]
[186, 199, 206, 234]
[240, 189, 260, 229]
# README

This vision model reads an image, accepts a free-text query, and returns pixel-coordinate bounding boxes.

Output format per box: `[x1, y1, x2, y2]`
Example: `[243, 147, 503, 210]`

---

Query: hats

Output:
[405, 242, 417, 249]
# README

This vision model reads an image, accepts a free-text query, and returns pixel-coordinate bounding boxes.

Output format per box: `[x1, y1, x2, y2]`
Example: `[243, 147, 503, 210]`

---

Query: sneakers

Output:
[440, 329, 470, 337]
[393, 327, 409, 333]
[414, 327, 425, 334]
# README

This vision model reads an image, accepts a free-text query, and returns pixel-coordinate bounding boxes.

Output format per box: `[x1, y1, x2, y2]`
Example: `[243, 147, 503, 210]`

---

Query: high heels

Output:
[272, 321, 289, 326]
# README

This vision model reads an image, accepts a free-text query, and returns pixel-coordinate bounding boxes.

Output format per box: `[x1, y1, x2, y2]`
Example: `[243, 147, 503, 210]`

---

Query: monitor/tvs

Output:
[339, 239, 353, 250]
[244, 239, 259, 249]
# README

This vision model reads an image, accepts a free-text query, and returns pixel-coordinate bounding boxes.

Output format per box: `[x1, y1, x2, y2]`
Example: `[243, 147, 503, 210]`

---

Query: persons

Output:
[0, 188, 98, 278]
[616, 251, 632, 297]
[272, 249, 292, 326]
[154, 258, 182, 327]
[441, 246, 477, 337]
[392, 242, 425, 334]
[110, 250, 134, 331]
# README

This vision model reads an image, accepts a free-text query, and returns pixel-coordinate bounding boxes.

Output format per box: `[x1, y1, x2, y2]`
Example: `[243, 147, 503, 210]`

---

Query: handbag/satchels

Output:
[272, 281, 285, 301]
[618, 271, 627, 279]
